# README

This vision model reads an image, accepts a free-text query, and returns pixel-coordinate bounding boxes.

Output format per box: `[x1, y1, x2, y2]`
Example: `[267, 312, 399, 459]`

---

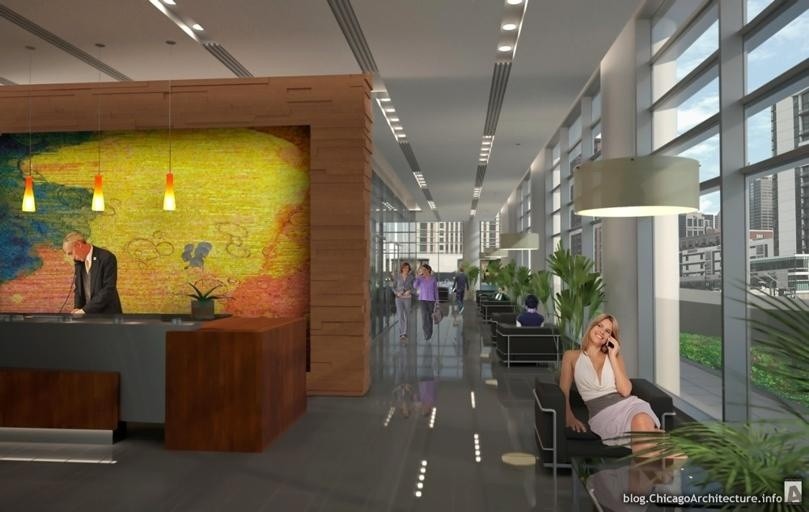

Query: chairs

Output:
[532, 378, 677, 478]
[476, 290, 563, 368]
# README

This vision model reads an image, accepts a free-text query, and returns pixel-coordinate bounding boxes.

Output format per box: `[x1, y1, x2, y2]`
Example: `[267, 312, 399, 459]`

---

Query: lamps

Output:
[91, 43, 106, 212]
[22, 46, 36, 214]
[500, 232, 539, 251]
[163, 41, 177, 211]
[574, 80, 700, 219]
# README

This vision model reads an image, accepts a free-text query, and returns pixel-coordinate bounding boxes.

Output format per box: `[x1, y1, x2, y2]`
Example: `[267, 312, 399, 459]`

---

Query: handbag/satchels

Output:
[397, 287, 412, 298]
[432, 302, 443, 325]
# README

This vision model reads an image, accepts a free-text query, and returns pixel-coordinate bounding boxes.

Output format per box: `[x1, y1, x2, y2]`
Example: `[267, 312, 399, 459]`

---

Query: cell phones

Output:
[606, 339, 610, 347]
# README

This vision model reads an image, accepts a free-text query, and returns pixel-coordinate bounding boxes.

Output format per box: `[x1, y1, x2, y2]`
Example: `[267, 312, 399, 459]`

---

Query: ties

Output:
[496, 293, 502, 301]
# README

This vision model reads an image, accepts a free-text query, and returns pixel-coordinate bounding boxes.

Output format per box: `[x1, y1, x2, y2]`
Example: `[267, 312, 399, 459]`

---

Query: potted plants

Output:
[185, 282, 223, 321]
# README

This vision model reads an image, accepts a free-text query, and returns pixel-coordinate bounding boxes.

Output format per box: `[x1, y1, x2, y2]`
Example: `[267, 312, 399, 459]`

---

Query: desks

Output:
[571, 456, 809, 512]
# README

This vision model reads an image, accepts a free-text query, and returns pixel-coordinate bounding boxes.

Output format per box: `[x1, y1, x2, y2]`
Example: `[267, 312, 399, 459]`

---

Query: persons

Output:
[451, 268, 470, 314]
[490, 287, 510, 302]
[557, 313, 694, 460]
[515, 295, 546, 329]
[62, 231, 123, 316]
[412, 264, 440, 340]
[418, 347, 436, 415]
[395, 348, 415, 417]
[391, 261, 416, 344]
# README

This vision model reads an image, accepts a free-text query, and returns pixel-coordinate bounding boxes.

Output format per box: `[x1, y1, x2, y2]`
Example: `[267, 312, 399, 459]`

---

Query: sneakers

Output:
[423, 332, 432, 341]
[400, 333, 408, 339]
[458, 305, 465, 314]
